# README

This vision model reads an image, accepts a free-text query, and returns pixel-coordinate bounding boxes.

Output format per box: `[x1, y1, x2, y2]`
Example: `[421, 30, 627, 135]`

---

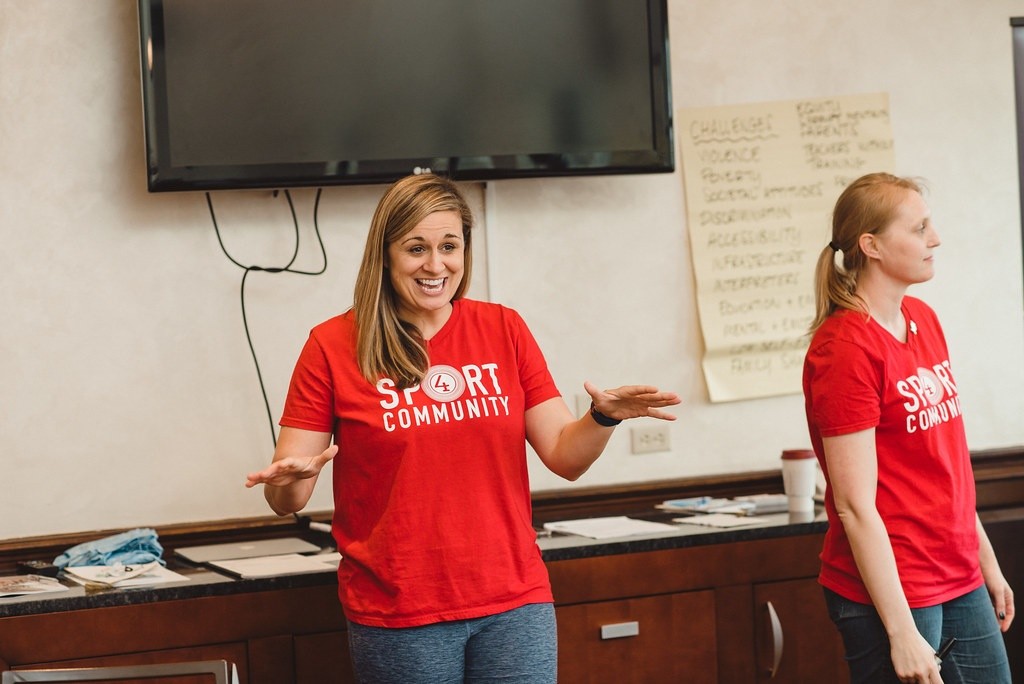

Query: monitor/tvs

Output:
[138, 0, 676, 192]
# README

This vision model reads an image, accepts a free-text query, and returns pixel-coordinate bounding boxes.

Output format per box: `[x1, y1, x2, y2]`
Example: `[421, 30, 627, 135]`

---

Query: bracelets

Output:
[590, 401, 623, 426]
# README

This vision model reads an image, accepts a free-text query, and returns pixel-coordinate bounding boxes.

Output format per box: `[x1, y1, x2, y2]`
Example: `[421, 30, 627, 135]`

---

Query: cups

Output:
[781, 450, 818, 513]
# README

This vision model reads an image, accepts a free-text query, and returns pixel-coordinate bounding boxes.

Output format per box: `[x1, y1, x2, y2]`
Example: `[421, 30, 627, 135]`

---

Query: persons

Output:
[245, 176, 681, 682]
[802, 172, 1015, 684]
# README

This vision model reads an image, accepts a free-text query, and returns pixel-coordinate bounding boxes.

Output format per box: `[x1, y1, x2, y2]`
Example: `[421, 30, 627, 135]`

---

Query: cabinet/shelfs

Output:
[0, 508, 1024, 684]
